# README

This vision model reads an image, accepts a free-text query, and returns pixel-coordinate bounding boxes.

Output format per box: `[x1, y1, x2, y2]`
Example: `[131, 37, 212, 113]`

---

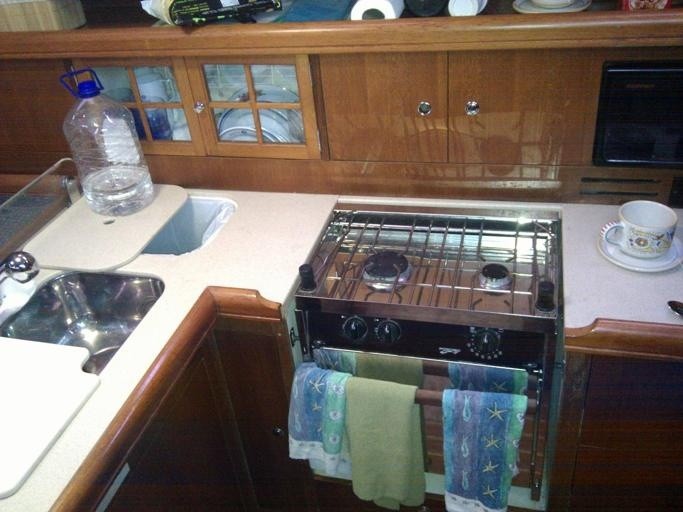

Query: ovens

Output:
[592, 61, 683, 169]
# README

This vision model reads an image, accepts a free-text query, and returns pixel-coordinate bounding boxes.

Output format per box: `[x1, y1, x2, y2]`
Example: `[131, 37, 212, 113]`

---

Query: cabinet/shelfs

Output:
[1, 21, 322, 193]
[308, 10, 607, 205]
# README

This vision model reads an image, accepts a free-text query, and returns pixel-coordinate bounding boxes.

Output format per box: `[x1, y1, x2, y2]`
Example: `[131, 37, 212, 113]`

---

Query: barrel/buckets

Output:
[59, 67, 154, 215]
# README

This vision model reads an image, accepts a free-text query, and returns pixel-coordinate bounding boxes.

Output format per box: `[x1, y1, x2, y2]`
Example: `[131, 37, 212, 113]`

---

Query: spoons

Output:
[667, 300, 683, 317]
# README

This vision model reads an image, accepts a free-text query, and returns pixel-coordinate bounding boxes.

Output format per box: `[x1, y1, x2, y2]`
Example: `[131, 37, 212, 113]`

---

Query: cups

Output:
[600, 200, 678, 259]
[103, 74, 176, 141]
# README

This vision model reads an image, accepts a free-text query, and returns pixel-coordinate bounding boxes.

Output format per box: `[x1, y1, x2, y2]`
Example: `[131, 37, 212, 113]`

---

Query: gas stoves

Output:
[295, 204, 562, 372]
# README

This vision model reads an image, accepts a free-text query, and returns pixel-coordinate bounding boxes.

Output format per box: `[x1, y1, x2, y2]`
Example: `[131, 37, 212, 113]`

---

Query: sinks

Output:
[0, 269, 165, 374]
[140, 196, 241, 255]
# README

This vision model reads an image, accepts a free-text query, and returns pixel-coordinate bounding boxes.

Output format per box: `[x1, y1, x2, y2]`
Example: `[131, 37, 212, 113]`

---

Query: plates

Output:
[513, 0, 593, 15]
[217, 83, 305, 143]
[598, 236, 682, 272]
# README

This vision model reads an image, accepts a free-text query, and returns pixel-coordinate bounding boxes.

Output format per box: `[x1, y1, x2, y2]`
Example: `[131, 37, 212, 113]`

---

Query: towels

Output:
[441, 388, 528, 512]
[447, 360, 530, 396]
[344, 375, 426, 511]
[286, 361, 351, 482]
[355, 349, 425, 388]
[311, 346, 356, 375]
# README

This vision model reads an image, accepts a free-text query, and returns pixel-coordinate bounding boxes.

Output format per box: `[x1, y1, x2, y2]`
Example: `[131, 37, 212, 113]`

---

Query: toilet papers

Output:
[350, 0, 405, 21]
